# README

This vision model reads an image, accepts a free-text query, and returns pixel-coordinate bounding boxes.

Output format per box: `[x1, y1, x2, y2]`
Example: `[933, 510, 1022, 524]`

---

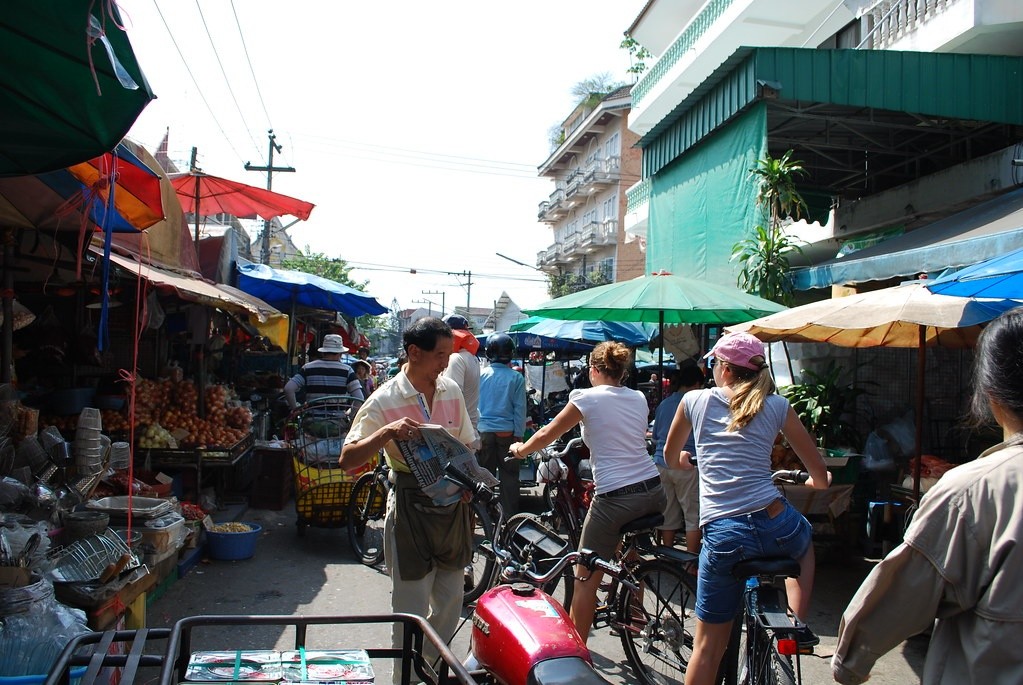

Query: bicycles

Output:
[285, 386, 578, 617]
[504, 435, 730, 685]
[687, 454, 821, 685]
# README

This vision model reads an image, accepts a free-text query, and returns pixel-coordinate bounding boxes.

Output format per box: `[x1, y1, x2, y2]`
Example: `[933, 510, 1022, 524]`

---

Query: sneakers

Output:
[796, 628, 820, 648]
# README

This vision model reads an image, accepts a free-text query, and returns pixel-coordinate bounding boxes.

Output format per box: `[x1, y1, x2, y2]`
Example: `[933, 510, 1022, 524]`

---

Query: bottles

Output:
[744, 578, 760, 615]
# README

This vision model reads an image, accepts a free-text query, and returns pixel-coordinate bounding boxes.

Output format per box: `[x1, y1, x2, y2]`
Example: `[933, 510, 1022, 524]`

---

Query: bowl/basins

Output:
[66, 510, 110, 539]
[205, 522, 262, 561]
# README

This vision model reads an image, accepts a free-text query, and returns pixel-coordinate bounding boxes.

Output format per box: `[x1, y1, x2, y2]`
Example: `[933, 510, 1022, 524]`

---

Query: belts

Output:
[602, 476, 662, 497]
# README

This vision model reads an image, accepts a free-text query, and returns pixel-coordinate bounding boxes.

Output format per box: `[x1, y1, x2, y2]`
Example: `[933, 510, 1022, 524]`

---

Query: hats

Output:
[441, 314, 476, 332]
[702, 332, 766, 372]
[318, 334, 350, 353]
[350, 360, 371, 374]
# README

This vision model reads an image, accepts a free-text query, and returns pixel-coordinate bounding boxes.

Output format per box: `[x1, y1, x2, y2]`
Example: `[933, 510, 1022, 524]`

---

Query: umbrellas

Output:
[0, 0, 392, 377]
[484, 245, 1023, 508]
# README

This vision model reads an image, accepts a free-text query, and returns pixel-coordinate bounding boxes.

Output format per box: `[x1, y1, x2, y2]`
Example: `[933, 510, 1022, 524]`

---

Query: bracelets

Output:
[515, 449, 524, 458]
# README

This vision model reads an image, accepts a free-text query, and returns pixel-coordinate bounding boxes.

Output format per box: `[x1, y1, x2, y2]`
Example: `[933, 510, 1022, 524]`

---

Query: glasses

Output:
[710, 359, 733, 371]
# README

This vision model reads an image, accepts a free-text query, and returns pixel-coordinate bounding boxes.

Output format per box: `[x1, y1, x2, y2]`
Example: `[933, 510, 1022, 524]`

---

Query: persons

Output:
[509, 341, 667, 651]
[389, 359, 408, 377]
[443, 314, 528, 545]
[652, 363, 708, 575]
[832, 310, 1023, 685]
[285, 334, 378, 438]
[340, 316, 476, 685]
[661, 331, 832, 685]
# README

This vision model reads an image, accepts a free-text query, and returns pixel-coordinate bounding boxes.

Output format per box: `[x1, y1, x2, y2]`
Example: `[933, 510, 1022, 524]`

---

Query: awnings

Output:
[83, 237, 282, 323]
[780, 188, 1023, 294]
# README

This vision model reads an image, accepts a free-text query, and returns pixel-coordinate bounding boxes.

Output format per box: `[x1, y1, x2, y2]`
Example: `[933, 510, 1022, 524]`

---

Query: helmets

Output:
[484, 333, 515, 363]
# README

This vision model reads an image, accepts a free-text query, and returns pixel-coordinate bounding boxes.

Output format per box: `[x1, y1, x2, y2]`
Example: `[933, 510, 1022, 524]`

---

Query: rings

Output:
[409, 431, 414, 437]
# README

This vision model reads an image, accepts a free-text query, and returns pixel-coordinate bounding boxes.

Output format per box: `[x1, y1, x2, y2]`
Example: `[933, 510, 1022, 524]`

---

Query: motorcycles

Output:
[443, 461, 640, 685]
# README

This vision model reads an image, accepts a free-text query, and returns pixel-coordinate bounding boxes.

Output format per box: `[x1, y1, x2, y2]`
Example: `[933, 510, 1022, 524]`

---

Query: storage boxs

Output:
[248, 445, 293, 511]
[110, 495, 208, 609]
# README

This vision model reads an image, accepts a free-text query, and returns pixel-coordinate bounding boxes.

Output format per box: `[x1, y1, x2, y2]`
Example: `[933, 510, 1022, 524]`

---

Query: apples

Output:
[37, 379, 252, 450]
